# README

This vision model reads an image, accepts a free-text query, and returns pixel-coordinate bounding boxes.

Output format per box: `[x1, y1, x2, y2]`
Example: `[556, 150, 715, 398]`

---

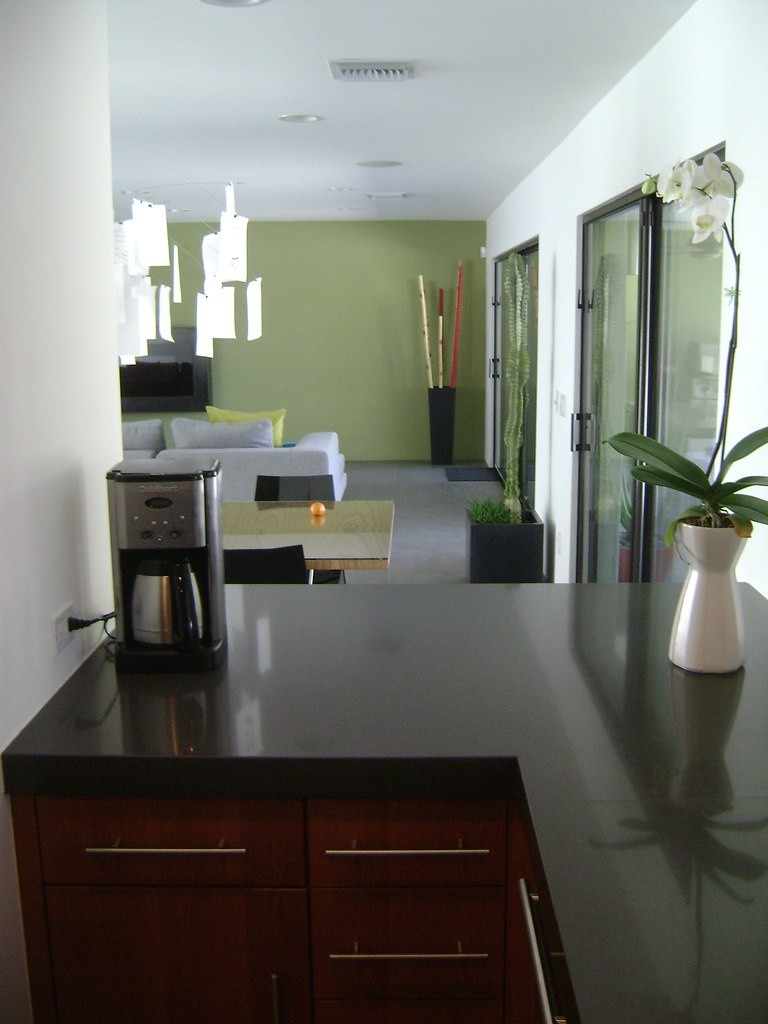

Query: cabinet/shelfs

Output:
[1, 582, 768, 1024]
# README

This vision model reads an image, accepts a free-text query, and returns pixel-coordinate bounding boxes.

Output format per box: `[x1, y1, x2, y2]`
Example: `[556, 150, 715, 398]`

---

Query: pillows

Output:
[121, 419, 167, 453]
[171, 418, 274, 448]
[205, 406, 286, 448]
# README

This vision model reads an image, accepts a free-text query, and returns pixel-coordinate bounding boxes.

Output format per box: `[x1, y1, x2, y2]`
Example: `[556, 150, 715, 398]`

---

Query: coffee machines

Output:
[106, 457, 228, 673]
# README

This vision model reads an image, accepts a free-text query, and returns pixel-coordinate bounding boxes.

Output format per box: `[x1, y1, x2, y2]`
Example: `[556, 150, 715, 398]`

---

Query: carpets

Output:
[445, 466, 500, 481]
[525, 459, 536, 481]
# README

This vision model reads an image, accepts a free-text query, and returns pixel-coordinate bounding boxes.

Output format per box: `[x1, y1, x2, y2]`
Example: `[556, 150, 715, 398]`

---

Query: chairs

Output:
[223, 545, 310, 584]
[254, 474, 346, 584]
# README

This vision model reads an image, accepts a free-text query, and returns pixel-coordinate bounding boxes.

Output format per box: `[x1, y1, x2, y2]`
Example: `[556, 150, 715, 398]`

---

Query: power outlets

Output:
[51, 602, 76, 654]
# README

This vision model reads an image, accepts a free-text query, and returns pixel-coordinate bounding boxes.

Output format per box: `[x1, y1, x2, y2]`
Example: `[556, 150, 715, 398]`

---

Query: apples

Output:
[310, 501, 325, 516]
[311, 515, 325, 527]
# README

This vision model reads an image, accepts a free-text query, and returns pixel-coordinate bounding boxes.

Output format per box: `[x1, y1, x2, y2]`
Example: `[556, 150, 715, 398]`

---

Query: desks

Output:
[218, 500, 396, 585]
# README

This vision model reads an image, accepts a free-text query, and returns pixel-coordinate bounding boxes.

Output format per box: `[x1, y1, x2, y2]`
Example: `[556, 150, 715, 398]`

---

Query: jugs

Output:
[129, 556, 203, 651]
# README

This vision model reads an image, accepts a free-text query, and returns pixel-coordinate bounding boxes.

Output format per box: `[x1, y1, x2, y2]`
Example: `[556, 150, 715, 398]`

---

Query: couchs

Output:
[122, 432, 348, 504]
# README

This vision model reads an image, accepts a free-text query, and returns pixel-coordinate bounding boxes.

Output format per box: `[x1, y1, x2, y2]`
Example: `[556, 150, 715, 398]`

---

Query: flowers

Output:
[601, 151, 768, 548]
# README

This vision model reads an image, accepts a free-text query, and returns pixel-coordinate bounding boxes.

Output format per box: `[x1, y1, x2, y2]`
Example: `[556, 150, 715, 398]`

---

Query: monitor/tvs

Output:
[119, 326, 213, 414]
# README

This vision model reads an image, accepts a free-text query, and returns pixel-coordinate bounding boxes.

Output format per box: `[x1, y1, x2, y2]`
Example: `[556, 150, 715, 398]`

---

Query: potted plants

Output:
[463, 252, 544, 585]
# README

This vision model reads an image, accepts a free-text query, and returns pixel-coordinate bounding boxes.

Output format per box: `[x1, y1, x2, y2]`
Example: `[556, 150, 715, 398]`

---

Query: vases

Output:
[428, 385, 456, 466]
[668, 514, 749, 674]
